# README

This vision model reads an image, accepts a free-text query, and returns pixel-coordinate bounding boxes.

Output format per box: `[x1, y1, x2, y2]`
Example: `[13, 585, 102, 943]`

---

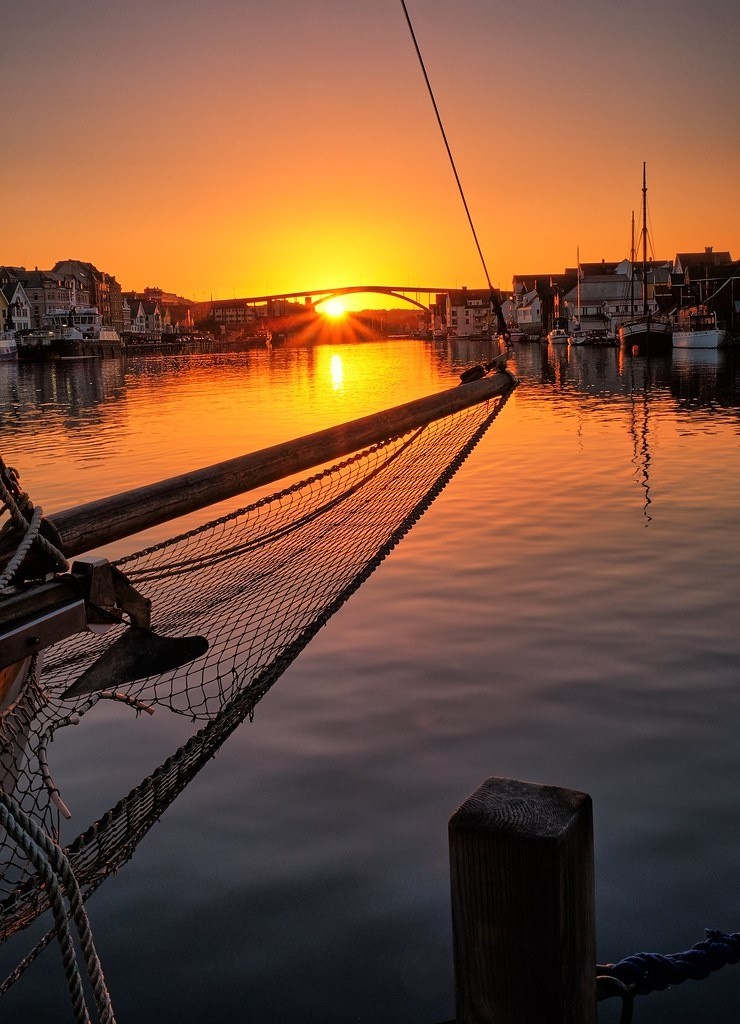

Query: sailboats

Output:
[0, 0, 523, 1024]
[547, 161, 732, 349]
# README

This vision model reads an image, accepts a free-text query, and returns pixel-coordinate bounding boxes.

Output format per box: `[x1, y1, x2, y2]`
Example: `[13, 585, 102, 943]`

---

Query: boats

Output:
[432, 329, 442, 340]
[490, 332, 509, 341]
[469, 333, 484, 341]
[500, 328, 527, 342]
[446, 331, 471, 340]
[526, 334, 542, 342]
[12, 291, 122, 360]
[0, 325, 18, 361]
